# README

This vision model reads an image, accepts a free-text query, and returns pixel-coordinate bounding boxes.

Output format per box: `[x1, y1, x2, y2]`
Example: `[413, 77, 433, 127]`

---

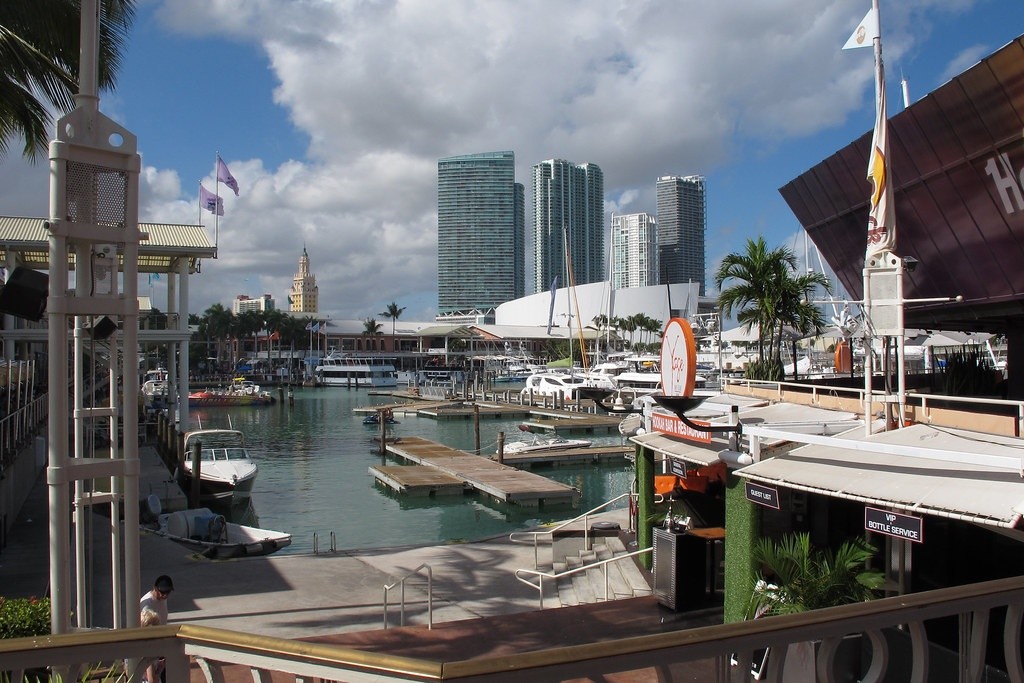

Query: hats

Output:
[155, 575, 174, 592]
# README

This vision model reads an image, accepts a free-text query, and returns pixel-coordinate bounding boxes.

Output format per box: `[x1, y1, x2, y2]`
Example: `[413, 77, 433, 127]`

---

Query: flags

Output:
[199, 183, 224, 216]
[303, 321, 312, 331]
[218, 156, 240, 197]
[316, 322, 326, 334]
[312, 322, 321, 334]
[864, 60, 895, 263]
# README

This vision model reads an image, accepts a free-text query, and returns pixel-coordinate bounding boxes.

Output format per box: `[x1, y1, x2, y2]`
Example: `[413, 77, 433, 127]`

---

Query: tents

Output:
[696, 311, 1006, 387]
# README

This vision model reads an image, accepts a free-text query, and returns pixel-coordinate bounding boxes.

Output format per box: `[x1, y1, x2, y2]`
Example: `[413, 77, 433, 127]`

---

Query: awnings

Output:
[627, 403, 860, 466]
[730, 407, 1024, 530]
[617, 394, 770, 437]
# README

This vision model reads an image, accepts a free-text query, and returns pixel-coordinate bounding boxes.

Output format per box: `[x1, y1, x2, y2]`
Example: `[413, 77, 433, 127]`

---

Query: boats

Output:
[496, 425, 593, 455]
[495, 225, 709, 399]
[187, 377, 273, 407]
[315, 350, 399, 388]
[142, 369, 169, 406]
[121, 495, 293, 557]
[177, 413, 259, 504]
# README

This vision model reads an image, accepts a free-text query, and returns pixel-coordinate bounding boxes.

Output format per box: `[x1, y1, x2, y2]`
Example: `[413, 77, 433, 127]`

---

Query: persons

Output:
[123, 609, 167, 683]
[374, 407, 394, 421]
[138, 575, 176, 683]
[154, 372, 262, 396]
